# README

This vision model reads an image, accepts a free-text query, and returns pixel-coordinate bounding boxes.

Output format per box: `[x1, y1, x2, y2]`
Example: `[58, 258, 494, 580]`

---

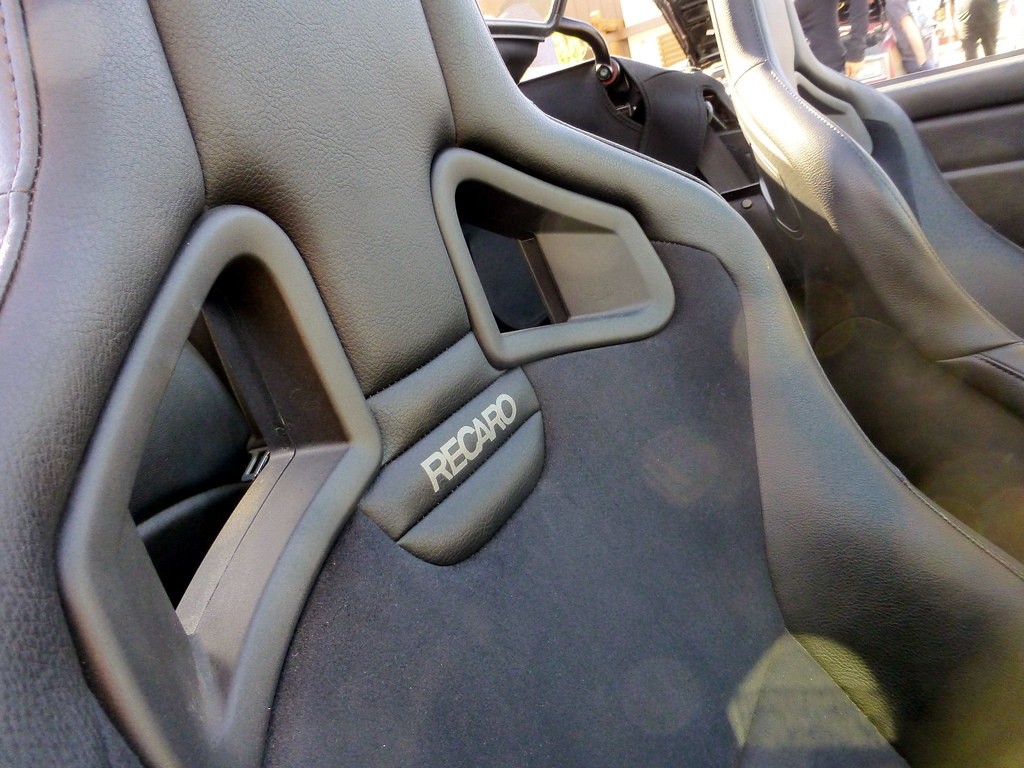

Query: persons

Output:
[794, 0, 869, 77]
[957, 0, 1000, 60]
[884, 0, 948, 74]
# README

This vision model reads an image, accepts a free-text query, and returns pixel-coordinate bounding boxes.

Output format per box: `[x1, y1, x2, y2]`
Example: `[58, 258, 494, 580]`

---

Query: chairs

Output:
[0, 0, 1024, 768]
[705, 0, 1024, 563]
